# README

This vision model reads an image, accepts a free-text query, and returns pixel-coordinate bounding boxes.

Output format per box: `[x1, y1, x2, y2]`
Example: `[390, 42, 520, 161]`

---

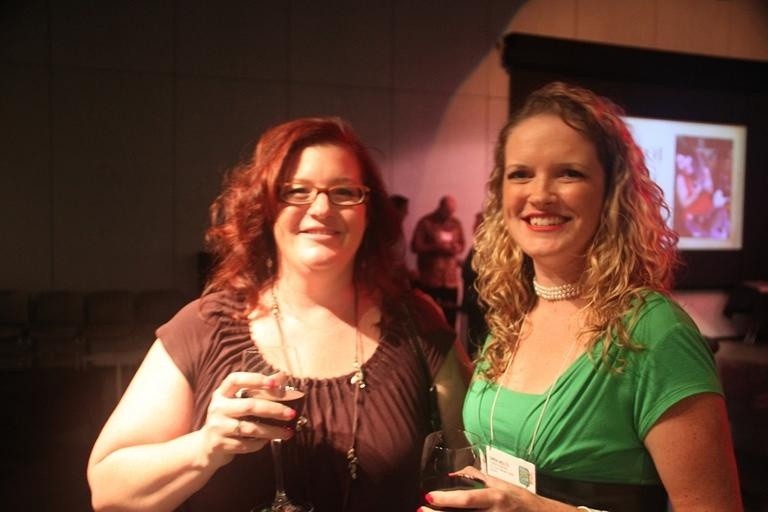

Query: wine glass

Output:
[240, 345, 315, 510]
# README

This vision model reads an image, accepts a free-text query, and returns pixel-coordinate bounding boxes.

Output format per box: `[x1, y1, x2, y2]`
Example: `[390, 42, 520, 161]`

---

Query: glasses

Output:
[272, 179, 370, 208]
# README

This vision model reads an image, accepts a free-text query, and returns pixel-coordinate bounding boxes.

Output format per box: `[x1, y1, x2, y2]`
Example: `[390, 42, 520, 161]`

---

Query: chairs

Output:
[1, 290, 194, 377]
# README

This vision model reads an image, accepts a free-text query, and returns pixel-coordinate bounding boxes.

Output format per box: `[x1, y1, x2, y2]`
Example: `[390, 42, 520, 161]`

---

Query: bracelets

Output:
[578, 506, 609, 512]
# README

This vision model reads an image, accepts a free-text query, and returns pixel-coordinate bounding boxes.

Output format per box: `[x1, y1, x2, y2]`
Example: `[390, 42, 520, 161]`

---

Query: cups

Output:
[422, 429, 486, 509]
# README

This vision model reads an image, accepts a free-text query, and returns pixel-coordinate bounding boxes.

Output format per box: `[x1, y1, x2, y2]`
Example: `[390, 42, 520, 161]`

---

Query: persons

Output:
[459, 212, 483, 353]
[412, 83, 744, 511]
[389, 193, 409, 282]
[681, 185, 730, 239]
[85, 115, 474, 511]
[411, 193, 466, 328]
[676, 153, 713, 221]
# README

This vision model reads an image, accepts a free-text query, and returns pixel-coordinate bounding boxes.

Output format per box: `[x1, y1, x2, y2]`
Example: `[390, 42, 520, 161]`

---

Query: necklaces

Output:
[269, 277, 366, 510]
[532, 260, 598, 299]
